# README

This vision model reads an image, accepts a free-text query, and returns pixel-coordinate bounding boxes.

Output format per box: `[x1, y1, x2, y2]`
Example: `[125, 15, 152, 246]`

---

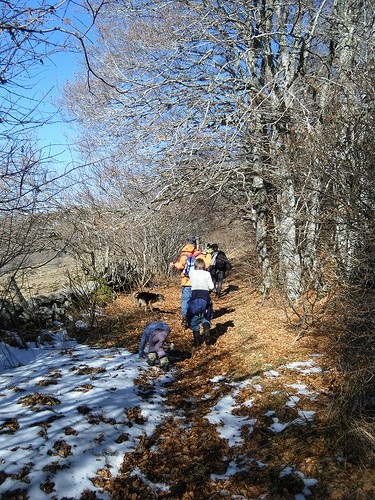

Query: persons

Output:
[169, 238, 232, 328]
[188, 258, 215, 347]
[139, 321, 171, 371]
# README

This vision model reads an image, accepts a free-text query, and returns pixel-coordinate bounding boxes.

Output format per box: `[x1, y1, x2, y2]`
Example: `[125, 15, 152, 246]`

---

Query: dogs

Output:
[133, 291, 166, 313]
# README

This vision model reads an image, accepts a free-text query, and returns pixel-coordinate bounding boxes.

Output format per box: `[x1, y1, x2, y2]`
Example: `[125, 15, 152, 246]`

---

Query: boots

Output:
[203, 322, 212, 345]
[193, 330, 200, 348]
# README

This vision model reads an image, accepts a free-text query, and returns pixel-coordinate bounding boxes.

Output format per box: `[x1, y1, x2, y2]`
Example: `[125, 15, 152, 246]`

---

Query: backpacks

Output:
[182, 252, 202, 276]
[216, 251, 225, 271]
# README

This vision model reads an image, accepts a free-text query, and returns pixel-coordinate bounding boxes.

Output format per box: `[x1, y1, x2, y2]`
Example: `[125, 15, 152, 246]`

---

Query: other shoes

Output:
[216, 292, 220, 298]
[163, 365, 169, 372]
[147, 359, 155, 366]
[181, 319, 190, 328]
